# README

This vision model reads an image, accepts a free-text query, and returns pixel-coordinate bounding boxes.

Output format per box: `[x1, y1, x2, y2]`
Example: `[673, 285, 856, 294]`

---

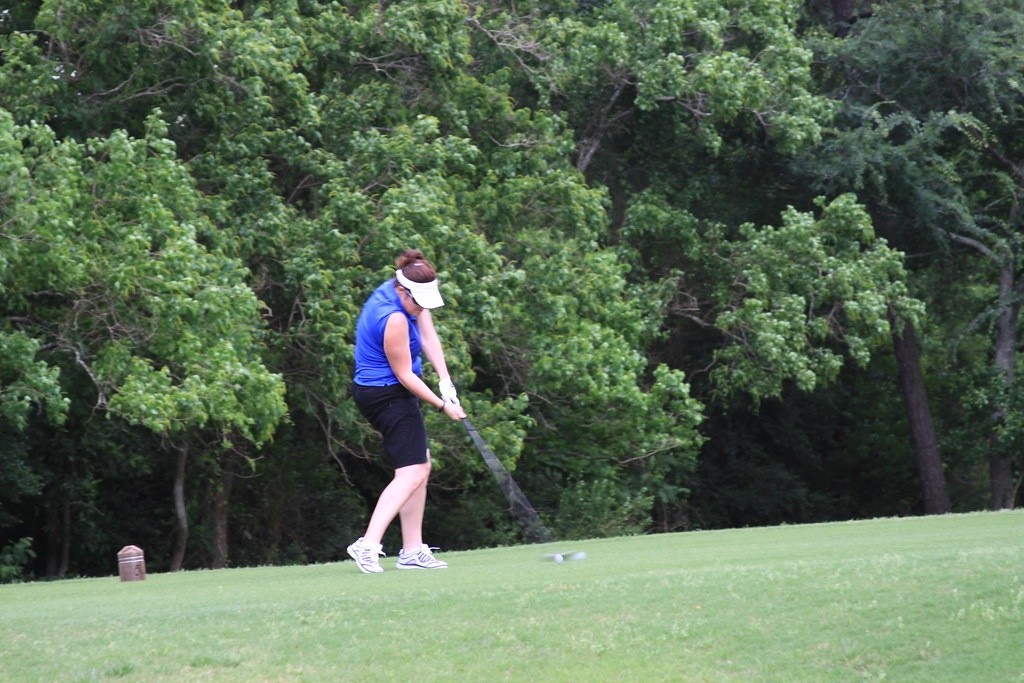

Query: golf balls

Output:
[554, 553, 564, 564]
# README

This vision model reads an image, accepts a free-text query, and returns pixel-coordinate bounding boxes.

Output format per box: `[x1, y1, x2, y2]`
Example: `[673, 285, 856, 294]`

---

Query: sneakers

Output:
[346, 537, 387, 574]
[395, 544, 448, 569]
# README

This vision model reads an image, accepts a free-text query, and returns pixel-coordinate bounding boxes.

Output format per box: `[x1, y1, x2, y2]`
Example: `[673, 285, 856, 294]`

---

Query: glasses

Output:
[404, 288, 418, 305]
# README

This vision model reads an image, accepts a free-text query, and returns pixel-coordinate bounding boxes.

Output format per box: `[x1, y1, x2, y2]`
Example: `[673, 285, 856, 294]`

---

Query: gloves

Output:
[438, 377, 461, 407]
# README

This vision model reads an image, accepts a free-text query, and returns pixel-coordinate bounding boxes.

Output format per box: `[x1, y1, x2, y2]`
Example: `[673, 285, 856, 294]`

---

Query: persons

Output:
[347, 248, 467, 574]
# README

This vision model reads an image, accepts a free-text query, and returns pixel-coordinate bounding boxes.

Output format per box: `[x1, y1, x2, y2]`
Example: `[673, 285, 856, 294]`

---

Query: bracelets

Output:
[439, 401, 445, 413]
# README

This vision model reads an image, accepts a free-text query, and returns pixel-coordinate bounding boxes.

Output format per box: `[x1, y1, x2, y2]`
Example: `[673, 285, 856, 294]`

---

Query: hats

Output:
[395, 269, 445, 309]
